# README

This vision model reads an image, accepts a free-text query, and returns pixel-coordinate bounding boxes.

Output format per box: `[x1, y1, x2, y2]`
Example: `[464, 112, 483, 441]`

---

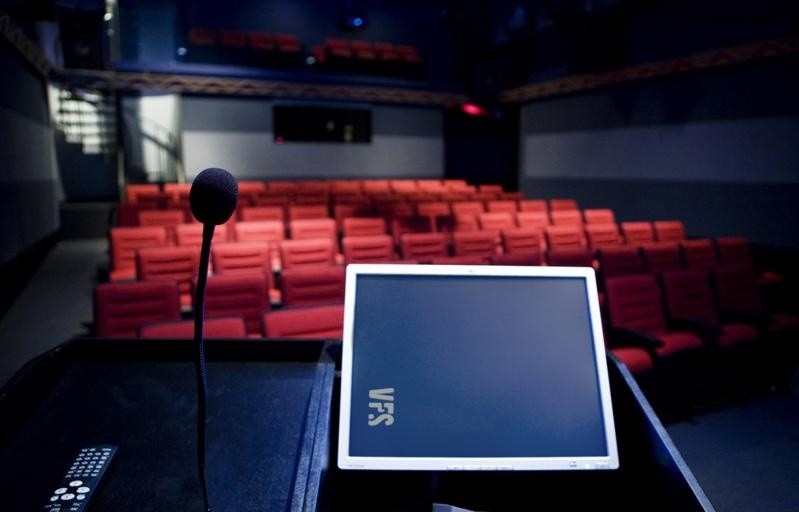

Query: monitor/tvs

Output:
[336, 263, 620, 471]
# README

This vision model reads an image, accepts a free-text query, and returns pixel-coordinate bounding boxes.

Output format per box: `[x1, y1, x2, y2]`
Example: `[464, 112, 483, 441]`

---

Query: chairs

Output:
[90, 176, 799, 429]
[186, 29, 420, 78]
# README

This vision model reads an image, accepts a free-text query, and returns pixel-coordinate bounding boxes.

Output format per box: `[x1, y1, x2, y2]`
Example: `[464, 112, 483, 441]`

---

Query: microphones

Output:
[188, 167, 239, 512]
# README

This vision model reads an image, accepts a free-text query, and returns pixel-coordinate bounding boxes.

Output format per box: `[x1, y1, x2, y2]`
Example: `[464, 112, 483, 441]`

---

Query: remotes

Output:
[40, 444, 120, 512]
